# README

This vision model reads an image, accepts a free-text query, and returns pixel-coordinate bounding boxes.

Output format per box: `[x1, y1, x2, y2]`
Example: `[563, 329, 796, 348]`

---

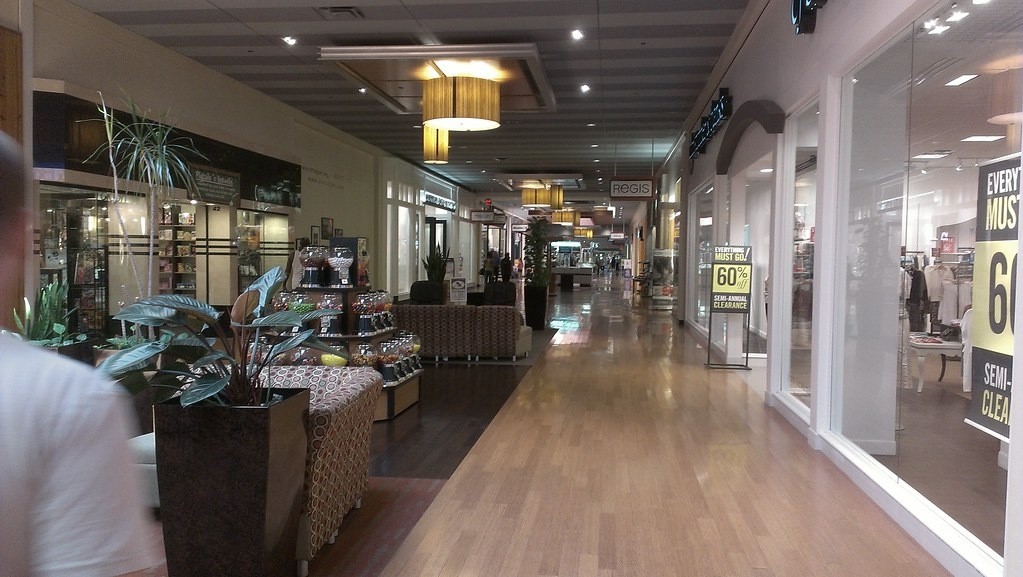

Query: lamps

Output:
[423, 76, 501, 132]
[424, 123, 448, 165]
[522, 187, 593, 237]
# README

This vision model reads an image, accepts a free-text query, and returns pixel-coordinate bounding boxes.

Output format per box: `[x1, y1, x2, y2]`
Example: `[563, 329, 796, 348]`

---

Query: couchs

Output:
[112, 364, 383, 577]
[392, 280, 455, 305]
[392, 305, 532, 361]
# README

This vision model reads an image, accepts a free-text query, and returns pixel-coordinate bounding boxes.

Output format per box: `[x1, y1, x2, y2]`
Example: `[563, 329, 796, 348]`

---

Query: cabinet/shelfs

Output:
[65, 203, 260, 346]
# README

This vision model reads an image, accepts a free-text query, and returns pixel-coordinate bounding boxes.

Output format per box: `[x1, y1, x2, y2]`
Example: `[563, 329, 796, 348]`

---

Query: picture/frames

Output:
[296, 216, 343, 248]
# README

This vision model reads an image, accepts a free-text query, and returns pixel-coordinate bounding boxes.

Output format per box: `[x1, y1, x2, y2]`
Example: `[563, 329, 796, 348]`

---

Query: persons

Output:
[598, 259, 605, 273]
[607, 258, 610, 264]
[0, 128, 169, 577]
[501, 252, 513, 281]
[611, 256, 619, 271]
[485, 249, 500, 284]
[517, 258, 523, 277]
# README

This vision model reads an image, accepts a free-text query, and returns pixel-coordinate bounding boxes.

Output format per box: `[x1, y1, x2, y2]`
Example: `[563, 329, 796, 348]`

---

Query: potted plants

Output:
[484, 257, 517, 307]
[96, 300, 155, 369]
[100, 266, 352, 577]
[1, 277, 93, 369]
[421, 241, 452, 305]
[524, 217, 550, 330]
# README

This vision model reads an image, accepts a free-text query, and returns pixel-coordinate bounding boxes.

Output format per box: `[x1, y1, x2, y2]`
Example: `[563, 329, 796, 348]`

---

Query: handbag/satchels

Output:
[478, 268, 484, 275]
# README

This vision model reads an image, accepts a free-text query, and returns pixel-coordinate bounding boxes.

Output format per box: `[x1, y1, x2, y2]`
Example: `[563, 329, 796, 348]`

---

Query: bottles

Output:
[564, 255, 568, 267]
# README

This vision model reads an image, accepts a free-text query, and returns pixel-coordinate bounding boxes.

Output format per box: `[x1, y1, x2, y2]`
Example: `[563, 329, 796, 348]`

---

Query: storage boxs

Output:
[330, 237, 372, 286]
